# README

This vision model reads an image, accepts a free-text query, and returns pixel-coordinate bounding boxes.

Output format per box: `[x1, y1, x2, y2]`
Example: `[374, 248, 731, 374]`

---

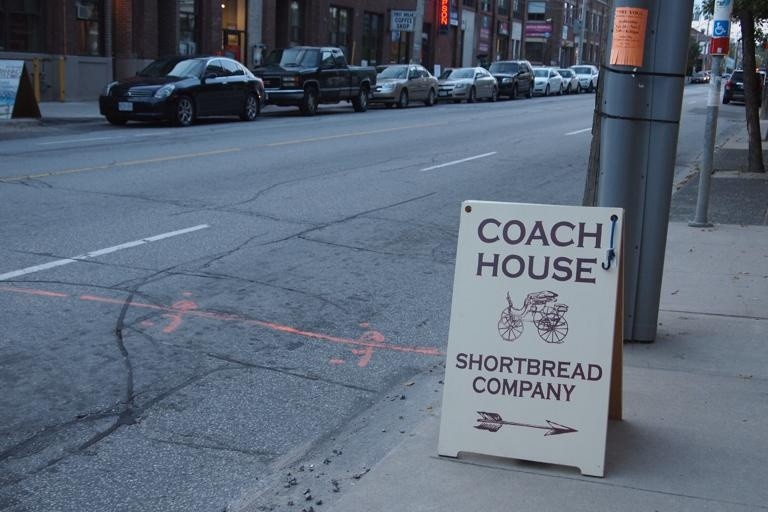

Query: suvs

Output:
[487, 57, 536, 101]
[723, 66, 767, 108]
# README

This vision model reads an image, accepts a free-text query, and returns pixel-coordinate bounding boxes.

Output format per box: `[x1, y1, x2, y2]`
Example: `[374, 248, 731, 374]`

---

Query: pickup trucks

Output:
[251, 44, 378, 116]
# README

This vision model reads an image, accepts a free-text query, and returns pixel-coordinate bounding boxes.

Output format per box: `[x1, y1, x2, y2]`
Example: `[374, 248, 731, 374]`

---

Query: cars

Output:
[370, 63, 440, 109]
[532, 67, 564, 97]
[98, 55, 266, 127]
[556, 69, 582, 95]
[570, 64, 599, 93]
[437, 66, 500, 103]
[690, 70, 712, 84]
